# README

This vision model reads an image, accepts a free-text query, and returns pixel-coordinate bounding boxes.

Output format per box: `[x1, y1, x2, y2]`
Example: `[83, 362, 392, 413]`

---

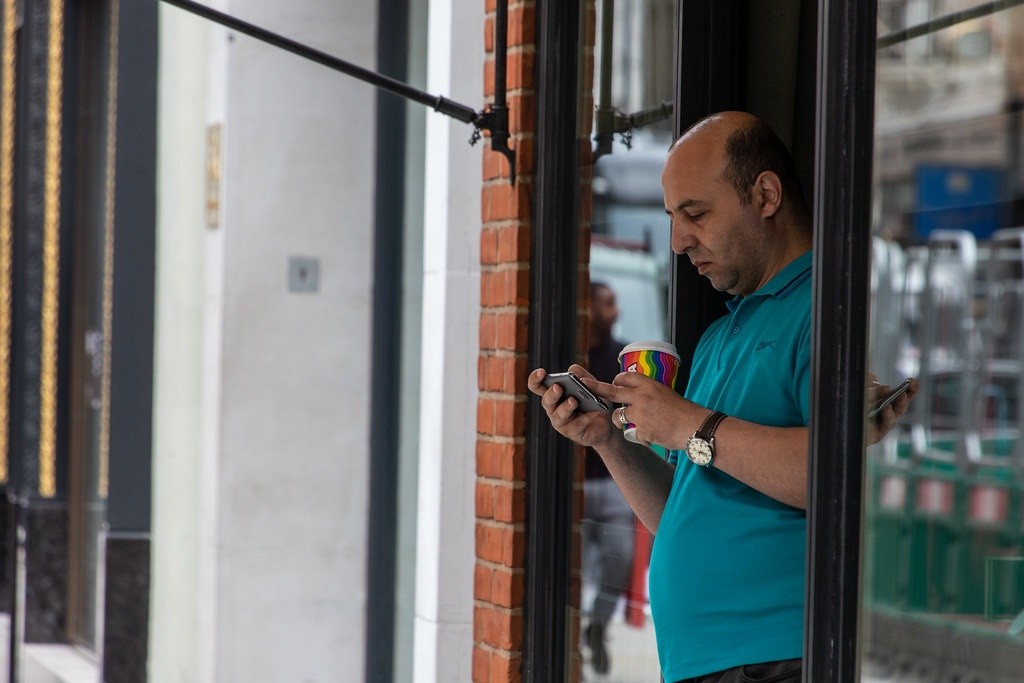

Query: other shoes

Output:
[583, 622, 610, 674]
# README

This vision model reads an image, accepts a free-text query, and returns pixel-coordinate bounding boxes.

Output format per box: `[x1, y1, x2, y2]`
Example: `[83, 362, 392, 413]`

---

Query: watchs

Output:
[685, 410, 728, 468]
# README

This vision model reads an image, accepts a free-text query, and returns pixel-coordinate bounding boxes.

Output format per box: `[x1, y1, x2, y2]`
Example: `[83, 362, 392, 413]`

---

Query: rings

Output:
[619, 407, 629, 424]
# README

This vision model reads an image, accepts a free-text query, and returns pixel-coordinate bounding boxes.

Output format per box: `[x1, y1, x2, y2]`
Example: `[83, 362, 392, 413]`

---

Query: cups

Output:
[617, 339, 682, 445]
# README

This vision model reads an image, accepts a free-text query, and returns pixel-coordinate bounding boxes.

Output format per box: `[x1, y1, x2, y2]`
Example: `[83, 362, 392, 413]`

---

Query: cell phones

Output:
[868, 378, 914, 419]
[543, 373, 608, 414]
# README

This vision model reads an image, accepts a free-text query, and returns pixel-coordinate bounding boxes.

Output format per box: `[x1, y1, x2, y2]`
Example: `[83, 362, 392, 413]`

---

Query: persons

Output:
[582, 284, 633, 673]
[868, 374, 918, 444]
[528, 111, 813, 683]
